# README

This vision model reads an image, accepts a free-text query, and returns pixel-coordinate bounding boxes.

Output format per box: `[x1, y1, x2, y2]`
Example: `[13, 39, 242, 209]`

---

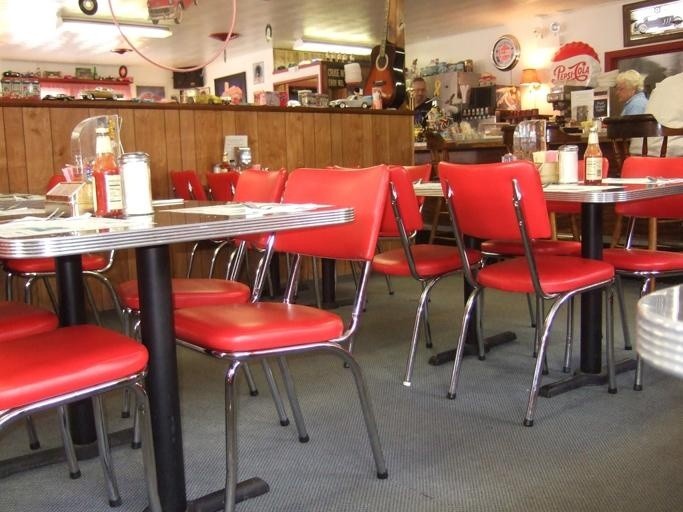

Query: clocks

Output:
[491, 34, 520, 72]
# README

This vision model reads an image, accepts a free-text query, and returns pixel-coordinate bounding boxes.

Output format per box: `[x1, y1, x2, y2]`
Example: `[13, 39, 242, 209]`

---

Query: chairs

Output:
[603, 155, 683, 391]
[0, 297, 61, 449]
[129, 164, 391, 512]
[435, 160, 619, 428]
[117, 169, 290, 448]
[424, 132, 456, 245]
[478, 158, 608, 327]
[202, 172, 276, 300]
[3, 167, 122, 320]
[170, 170, 229, 279]
[0, 323, 162, 511]
[344, 166, 486, 388]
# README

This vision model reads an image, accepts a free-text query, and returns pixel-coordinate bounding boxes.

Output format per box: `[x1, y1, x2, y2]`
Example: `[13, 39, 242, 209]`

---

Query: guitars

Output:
[364, 0, 406, 108]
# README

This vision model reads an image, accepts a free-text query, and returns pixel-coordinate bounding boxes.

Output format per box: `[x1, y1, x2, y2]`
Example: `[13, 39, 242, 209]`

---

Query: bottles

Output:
[222, 151, 229, 169]
[92, 117, 123, 219]
[558, 144, 579, 183]
[239, 147, 252, 168]
[583, 126, 602, 185]
[118, 152, 154, 215]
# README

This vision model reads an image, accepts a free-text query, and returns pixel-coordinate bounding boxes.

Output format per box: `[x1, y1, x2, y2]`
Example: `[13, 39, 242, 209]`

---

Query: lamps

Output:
[519, 69, 541, 91]
[59, 17, 173, 39]
[292, 38, 372, 56]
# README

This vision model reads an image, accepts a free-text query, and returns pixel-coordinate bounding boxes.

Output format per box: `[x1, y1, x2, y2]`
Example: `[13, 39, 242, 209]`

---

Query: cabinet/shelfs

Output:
[423, 72, 481, 109]
[0, 99, 416, 315]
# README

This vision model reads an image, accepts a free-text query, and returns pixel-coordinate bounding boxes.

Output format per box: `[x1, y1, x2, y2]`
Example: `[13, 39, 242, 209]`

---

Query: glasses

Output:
[616, 87, 625, 90]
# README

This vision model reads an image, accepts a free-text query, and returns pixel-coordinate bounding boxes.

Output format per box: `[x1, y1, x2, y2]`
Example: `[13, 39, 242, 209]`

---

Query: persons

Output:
[630, 73, 682, 158]
[412, 77, 432, 125]
[615, 69, 649, 114]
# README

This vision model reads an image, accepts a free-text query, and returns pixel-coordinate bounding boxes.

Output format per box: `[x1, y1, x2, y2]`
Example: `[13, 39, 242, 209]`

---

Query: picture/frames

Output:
[214, 72, 247, 104]
[604, 40, 682, 116]
[622, 0, 683, 47]
